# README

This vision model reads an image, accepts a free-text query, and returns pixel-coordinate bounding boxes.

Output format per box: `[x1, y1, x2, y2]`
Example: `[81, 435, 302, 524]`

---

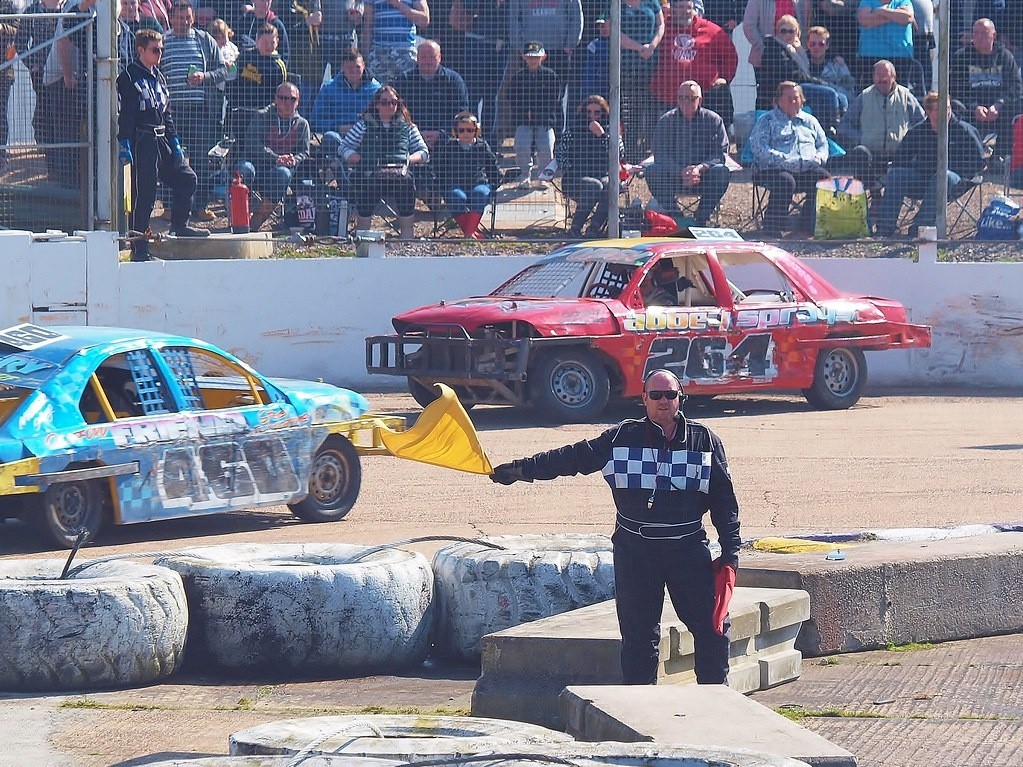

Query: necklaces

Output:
[646, 423, 676, 509]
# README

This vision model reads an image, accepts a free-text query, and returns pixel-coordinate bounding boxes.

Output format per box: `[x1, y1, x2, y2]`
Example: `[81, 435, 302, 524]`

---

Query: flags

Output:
[372, 382, 494, 475]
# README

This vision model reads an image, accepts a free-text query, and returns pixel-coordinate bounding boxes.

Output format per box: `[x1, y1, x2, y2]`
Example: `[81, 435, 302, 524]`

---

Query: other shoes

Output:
[539, 181, 549, 189]
[160, 210, 171, 222]
[192, 210, 216, 221]
[519, 180, 532, 188]
[584, 227, 602, 238]
[568, 227, 582, 239]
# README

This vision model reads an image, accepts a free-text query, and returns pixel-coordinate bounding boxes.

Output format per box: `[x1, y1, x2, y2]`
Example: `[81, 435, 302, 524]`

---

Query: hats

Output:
[523, 43, 545, 56]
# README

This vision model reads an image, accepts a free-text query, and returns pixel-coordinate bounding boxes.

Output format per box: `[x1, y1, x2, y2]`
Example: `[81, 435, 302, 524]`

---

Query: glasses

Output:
[459, 128, 474, 133]
[679, 95, 699, 102]
[586, 110, 601, 116]
[809, 42, 824, 47]
[276, 95, 297, 103]
[142, 47, 165, 54]
[645, 390, 681, 400]
[377, 99, 398, 107]
[780, 29, 795, 34]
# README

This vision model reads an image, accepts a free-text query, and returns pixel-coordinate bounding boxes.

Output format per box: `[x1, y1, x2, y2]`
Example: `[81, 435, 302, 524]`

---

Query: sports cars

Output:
[364, 210, 932, 421]
[0, 323, 369, 549]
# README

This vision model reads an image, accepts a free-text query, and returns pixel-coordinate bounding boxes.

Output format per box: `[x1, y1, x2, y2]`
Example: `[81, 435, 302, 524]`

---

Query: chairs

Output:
[208, 104, 1023, 233]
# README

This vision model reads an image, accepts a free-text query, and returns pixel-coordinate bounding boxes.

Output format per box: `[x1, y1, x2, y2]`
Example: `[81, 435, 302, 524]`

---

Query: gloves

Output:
[489, 457, 533, 485]
[718, 537, 741, 574]
[118, 139, 133, 165]
[170, 137, 184, 166]
[607, 285, 621, 298]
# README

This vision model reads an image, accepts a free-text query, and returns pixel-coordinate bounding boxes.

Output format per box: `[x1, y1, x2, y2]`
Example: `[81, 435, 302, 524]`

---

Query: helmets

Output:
[627, 261, 658, 284]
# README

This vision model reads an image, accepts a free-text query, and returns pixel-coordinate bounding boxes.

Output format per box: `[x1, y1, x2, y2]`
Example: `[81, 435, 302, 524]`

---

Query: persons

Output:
[115, 28, 211, 264]
[487, 368, 741, 690]
[0, 0, 1023, 236]
[607, 265, 678, 307]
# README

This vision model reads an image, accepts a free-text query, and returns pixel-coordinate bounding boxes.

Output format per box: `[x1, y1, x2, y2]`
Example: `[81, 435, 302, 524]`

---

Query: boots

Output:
[170, 213, 210, 237]
[130, 237, 162, 261]
[250, 198, 275, 232]
[355, 212, 485, 240]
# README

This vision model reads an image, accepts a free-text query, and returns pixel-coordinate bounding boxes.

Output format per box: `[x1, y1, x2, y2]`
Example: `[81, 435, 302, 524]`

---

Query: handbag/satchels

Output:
[814, 176, 872, 239]
[975, 196, 1021, 239]
